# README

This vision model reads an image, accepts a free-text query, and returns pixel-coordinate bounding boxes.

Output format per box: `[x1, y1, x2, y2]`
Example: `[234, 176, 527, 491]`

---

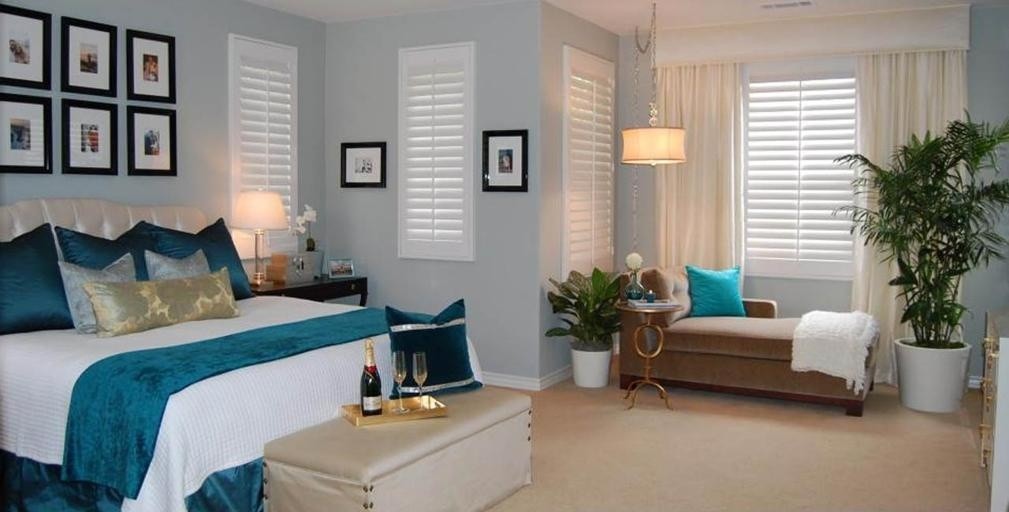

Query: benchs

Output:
[262, 384, 533, 511]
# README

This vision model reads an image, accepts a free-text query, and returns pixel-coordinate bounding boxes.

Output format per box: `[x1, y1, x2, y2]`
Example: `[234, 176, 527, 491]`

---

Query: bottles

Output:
[360, 337, 385, 414]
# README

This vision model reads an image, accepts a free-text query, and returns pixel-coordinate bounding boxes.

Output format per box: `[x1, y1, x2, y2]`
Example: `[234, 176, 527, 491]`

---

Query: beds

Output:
[0, 196, 484, 512]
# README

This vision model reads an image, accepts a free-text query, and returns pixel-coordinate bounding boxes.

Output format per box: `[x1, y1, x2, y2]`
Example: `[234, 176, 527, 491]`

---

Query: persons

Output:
[364, 160, 372, 173]
[502, 151, 511, 172]
[8, 40, 160, 156]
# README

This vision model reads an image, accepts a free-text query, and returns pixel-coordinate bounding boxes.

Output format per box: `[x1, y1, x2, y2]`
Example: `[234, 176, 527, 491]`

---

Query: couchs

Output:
[611, 264, 881, 416]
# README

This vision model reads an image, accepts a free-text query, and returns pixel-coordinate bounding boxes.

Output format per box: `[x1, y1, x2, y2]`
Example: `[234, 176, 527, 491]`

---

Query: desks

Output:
[613, 300, 685, 412]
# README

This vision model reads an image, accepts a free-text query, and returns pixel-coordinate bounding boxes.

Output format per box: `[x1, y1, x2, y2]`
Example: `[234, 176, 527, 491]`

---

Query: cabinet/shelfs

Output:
[979, 307, 1009, 511]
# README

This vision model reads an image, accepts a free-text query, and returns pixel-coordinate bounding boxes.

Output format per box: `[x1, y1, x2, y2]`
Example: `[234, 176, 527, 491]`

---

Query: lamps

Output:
[226, 190, 289, 288]
[617, 2, 689, 170]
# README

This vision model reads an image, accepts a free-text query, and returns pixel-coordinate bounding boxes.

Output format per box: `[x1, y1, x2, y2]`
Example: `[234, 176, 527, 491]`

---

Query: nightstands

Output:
[249, 275, 367, 308]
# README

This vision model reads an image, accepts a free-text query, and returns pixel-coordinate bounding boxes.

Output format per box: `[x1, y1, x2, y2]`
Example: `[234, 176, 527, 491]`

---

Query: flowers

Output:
[293, 203, 319, 238]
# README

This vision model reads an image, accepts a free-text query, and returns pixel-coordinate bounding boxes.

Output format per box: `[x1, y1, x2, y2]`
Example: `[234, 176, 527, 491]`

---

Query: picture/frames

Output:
[58, 15, 117, 98]
[61, 98, 118, 176]
[482, 128, 530, 192]
[124, 28, 178, 105]
[0, 4, 53, 91]
[339, 141, 386, 188]
[0, 93, 53, 174]
[125, 104, 178, 177]
[326, 257, 355, 278]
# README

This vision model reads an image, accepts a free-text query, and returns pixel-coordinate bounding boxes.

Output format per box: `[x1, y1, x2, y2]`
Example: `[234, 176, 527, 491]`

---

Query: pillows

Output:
[0, 222, 75, 335]
[684, 265, 747, 317]
[79, 267, 241, 339]
[383, 297, 483, 401]
[54, 220, 157, 281]
[146, 216, 255, 300]
[142, 248, 211, 281]
[56, 251, 136, 335]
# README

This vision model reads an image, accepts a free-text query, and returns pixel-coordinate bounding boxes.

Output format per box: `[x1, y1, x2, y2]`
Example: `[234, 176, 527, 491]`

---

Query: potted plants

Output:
[833, 108, 1007, 416]
[543, 266, 626, 389]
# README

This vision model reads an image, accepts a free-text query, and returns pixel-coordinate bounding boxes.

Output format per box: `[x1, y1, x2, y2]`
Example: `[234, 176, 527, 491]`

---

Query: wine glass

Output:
[412, 353, 428, 412]
[390, 348, 409, 414]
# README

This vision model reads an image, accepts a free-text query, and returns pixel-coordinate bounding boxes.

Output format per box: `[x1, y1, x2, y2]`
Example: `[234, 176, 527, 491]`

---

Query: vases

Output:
[305, 237, 315, 251]
[626, 272, 644, 299]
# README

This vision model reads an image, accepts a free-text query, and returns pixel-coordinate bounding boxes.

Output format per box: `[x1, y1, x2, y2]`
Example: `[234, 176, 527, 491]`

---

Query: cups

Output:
[647, 292, 656, 302]
